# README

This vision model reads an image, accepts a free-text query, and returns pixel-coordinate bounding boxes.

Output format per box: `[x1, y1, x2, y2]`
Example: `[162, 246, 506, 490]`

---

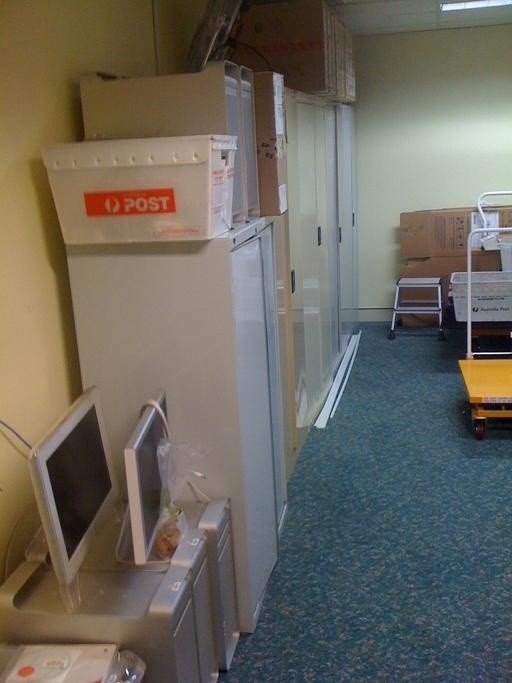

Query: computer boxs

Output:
[76, 60, 259, 230]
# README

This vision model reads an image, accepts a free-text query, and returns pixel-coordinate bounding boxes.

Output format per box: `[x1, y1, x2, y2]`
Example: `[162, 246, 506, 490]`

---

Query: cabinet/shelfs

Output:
[65, 219, 287, 632]
[280, 88, 355, 425]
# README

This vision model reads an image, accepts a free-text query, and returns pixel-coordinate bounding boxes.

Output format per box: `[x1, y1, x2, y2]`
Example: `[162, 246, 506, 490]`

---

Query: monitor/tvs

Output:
[27, 385, 115, 587]
[115, 390, 169, 571]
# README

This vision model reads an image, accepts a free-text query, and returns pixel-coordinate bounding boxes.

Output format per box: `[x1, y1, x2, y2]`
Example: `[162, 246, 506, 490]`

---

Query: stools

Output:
[388, 277, 444, 341]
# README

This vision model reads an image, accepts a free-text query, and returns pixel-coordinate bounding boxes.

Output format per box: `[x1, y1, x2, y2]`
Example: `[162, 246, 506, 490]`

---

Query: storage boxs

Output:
[398, 206, 512, 327]
[226, 0, 356, 216]
[40, 133, 238, 243]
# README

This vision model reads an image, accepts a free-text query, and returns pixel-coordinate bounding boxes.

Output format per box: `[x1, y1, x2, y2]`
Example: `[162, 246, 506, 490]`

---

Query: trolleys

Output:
[473, 189, 512, 351]
[455, 226, 512, 439]
